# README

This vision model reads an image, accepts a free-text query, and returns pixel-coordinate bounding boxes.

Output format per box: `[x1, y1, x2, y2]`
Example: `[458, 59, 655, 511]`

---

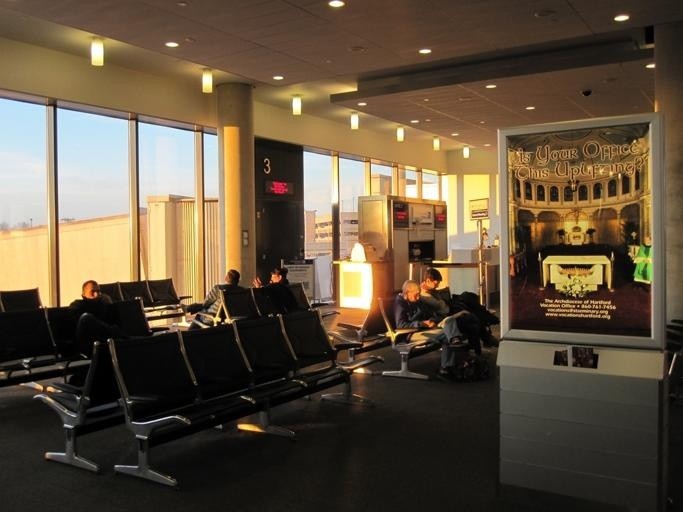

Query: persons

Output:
[252, 267, 289, 289]
[69, 280, 114, 308]
[393, 279, 471, 383]
[178, 268, 239, 330]
[419, 268, 498, 358]
[553, 347, 596, 368]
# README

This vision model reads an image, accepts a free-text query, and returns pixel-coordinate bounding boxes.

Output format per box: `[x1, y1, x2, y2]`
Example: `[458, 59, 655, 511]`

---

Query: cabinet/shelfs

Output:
[357, 196, 446, 301]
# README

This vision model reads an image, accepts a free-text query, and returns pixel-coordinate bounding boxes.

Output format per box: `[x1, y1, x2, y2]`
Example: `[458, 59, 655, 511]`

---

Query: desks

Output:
[332, 251, 500, 308]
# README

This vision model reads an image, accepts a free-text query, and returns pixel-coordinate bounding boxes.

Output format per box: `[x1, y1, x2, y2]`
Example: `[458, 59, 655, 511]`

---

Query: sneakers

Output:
[437, 367, 457, 384]
[449, 337, 467, 348]
[181, 303, 189, 313]
